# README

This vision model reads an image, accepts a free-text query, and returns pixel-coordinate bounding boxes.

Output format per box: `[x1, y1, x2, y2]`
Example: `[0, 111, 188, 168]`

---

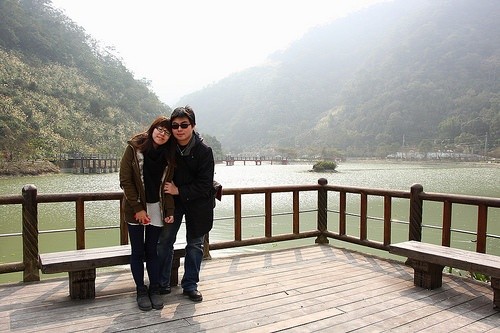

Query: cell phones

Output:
[134, 213, 150, 224]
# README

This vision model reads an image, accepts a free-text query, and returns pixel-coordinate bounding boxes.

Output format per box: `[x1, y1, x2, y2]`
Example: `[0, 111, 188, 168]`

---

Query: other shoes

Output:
[183, 289, 203, 301]
[161, 283, 171, 293]
[137, 284, 163, 310]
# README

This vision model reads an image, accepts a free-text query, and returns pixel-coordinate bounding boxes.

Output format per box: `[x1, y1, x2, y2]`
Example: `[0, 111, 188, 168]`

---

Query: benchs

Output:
[38, 243, 187, 301]
[389, 240, 500, 311]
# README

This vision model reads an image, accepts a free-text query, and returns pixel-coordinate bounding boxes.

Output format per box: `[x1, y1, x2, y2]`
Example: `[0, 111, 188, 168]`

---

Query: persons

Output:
[119, 116, 176, 311]
[163, 105, 215, 301]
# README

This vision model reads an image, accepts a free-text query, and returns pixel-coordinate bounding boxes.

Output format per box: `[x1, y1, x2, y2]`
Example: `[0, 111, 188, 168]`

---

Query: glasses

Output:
[155, 127, 171, 136]
[172, 123, 192, 129]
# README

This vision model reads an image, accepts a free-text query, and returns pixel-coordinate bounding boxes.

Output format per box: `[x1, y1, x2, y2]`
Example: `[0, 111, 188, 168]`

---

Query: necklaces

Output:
[177, 144, 188, 156]
